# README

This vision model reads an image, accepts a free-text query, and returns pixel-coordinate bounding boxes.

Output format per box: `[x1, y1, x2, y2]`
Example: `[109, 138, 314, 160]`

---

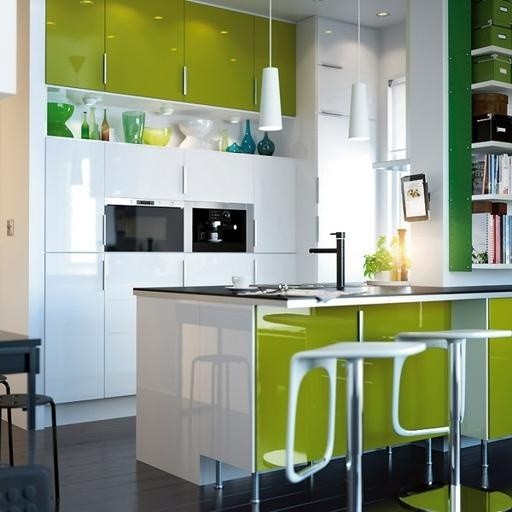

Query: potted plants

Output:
[362, 234, 396, 282]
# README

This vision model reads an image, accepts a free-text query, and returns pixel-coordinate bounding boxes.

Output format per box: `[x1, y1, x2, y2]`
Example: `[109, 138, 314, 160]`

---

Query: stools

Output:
[0, 393, 62, 511]
[0, 374, 15, 466]
[389, 329, 511, 512]
[264, 340, 427, 511]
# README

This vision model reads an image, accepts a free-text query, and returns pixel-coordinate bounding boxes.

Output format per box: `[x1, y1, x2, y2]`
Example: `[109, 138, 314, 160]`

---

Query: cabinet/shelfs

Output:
[452, 297, 511, 441]
[104, 0, 186, 104]
[186, 0, 256, 111]
[45, 0, 104, 93]
[199, 300, 451, 473]
[105, 141, 252, 204]
[256, 16, 296, 120]
[45, 134, 104, 251]
[46, 252, 105, 403]
[251, 153, 296, 254]
[255, 255, 296, 286]
[185, 252, 254, 286]
[278, 16, 377, 284]
[470, 46, 511, 270]
[104, 252, 184, 398]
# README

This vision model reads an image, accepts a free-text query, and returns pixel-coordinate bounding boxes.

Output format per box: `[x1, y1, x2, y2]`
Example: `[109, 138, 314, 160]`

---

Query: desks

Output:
[0, 329, 42, 468]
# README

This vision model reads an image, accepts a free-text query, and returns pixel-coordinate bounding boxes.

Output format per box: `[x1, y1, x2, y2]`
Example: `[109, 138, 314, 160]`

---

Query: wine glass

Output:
[178, 119, 213, 151]
[48, 102, 75, 137]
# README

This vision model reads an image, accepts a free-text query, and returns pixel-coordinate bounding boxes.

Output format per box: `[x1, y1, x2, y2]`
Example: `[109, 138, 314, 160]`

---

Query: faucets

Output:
[308, 230, 347, 289]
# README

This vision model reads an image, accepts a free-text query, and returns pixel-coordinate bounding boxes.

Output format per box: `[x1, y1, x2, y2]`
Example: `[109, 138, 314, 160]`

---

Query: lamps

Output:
[258, 0, 284, 132]
[346, 1, 371, 141]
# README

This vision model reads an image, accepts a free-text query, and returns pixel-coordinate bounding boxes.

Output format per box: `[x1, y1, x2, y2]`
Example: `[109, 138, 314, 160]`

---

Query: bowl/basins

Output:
[143, 126, 172, 146]
[364, 280, 410, 288]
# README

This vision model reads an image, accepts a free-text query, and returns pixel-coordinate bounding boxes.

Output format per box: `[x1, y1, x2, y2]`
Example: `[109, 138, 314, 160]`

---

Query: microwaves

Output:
[104, 198, 185, 252]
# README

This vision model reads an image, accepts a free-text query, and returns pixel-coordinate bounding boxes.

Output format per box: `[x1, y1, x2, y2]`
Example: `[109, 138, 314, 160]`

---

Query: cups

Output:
[220, 128, 228, 151]
[231, 275, 250, 288]
[122, 111, 145, 144]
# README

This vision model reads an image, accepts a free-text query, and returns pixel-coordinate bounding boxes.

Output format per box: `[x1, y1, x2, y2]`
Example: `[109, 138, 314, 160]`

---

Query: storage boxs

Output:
[472, 113, 511, 144]
[471, 25, 511, 50]
[470, 53, 511, 84]
[472, 0, 512, 30]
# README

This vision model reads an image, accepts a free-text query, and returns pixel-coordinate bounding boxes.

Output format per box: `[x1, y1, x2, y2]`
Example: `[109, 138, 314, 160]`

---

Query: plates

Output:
[226, 286, 258, 292]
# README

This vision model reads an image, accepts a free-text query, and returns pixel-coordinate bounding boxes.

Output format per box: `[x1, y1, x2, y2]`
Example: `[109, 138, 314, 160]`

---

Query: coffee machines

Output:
[198, 210, 238, 245]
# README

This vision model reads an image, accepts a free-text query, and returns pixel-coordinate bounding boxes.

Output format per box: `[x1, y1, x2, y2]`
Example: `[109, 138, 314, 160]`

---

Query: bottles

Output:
[391, 229, 408, 281]
[242, 119, 255, 154]
[226, 143, 243, 153]
[81, 107, 110, 141]
[257, 132, 275, 155]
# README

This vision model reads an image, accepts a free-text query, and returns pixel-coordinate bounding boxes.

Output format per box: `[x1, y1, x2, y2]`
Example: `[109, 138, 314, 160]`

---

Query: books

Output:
[471, 153, 512, 265]
